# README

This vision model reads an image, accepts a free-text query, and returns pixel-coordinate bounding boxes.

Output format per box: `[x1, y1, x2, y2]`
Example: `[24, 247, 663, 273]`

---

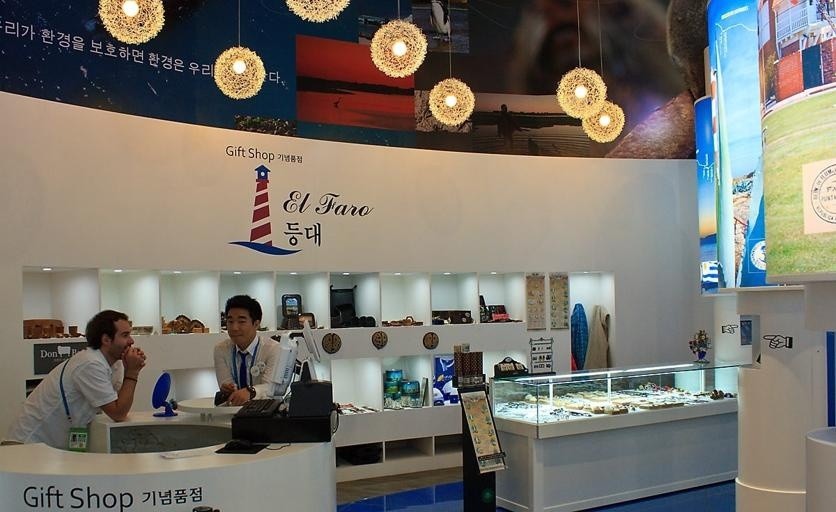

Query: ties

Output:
[235, 350, 249, 390]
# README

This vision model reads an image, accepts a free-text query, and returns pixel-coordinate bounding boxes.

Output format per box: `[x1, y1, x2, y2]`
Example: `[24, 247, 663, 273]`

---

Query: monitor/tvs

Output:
[302, 319, 321, 363]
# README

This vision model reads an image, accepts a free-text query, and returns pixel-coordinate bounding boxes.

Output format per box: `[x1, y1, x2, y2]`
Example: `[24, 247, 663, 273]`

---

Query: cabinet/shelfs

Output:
[489, 359, 751, 511]
[22, 270, 529, 479]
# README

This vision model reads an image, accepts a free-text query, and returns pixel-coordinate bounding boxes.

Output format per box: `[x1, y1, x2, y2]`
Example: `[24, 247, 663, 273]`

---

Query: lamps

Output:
[556, 68, 607, 119]
[582, 101, 624, 143]
[214, 45, 267, 100]
[97, 1, 166, 45]
[286, 1, 350, 23]
[370, 19, 428, 78]
[428, 77, 476, 126]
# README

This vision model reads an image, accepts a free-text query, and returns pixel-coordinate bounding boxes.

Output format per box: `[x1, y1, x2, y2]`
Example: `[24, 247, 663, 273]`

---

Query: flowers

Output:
[688, 329, 713, 363]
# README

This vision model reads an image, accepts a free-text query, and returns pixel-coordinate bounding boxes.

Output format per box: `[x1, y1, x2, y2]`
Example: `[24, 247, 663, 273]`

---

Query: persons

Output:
[213, 293, 283, 406]
[4, 309, 147, 451]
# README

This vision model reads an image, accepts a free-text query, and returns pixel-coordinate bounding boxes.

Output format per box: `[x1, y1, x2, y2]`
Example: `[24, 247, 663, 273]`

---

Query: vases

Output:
[698, 351, 706, 359]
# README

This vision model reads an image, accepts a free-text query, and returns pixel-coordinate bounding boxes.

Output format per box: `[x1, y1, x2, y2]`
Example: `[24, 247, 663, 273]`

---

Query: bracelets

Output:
[124, 376, 138, 382]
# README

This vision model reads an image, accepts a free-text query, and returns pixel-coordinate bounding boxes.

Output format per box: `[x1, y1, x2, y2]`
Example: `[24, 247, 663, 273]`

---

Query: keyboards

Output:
[235, 398, 284, 417]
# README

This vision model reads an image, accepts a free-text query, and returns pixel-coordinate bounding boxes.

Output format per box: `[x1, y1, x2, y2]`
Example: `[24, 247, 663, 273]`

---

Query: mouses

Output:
[224, 439, 253, 450]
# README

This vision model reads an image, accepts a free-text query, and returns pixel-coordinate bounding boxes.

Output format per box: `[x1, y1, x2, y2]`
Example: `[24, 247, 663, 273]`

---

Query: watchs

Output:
[245, 385, 256, 401]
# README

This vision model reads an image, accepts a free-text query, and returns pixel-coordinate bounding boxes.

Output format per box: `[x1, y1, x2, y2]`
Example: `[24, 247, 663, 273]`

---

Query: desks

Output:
[805, 428, 836, 512]
